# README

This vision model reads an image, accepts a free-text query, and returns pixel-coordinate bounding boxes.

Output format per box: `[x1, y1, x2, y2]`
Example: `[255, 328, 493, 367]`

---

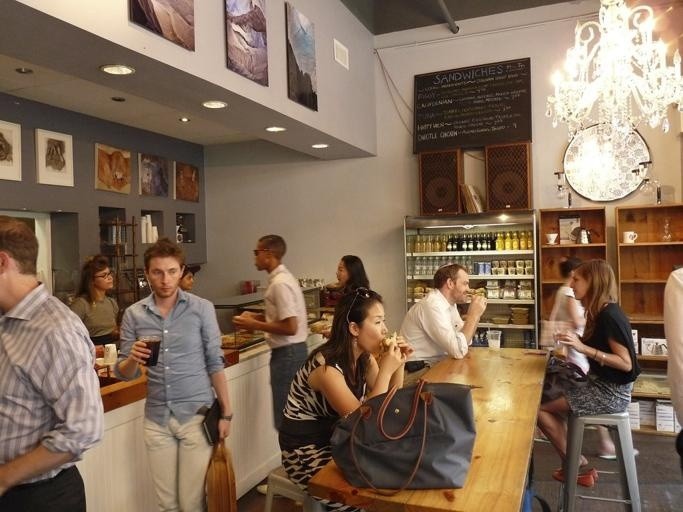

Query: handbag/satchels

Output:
[205, 438, 237, 512]
[331, 379, 476, 497]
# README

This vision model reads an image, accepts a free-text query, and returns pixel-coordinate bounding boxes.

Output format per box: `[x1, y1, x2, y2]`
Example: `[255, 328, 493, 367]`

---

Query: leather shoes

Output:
[552, 471, 595, 486]
[552, 464, 598, 480]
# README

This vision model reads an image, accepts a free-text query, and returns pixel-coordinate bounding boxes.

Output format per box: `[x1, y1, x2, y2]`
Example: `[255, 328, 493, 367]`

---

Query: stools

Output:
[559, 412, 642, 512]
[265, 467, 325, 512]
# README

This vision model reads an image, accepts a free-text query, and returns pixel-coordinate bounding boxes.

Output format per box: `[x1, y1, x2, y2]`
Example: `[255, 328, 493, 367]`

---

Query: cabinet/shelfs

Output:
[615, 205, 683, 438]
[403, 209, 538, 350]
[539, 206, 609, 320]
[213, 287, 321, 352]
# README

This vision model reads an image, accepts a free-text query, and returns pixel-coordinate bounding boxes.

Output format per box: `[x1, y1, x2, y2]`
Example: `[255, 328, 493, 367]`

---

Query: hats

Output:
[181, 265, 201, 279]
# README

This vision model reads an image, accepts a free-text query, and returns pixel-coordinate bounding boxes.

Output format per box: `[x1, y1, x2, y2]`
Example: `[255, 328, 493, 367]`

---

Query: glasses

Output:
[94, 271, 113, 280]
[346, 286, 369, 324]
[252, 248, 268, 257]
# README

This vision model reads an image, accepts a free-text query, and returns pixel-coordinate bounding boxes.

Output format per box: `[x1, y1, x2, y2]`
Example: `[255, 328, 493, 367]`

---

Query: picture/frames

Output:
[130, 0, 196, 54]
[173, 160, 200, 204]
[94, 144, 132, 196]
[0, 118, 21, 182]
[35, 128, 75, 187]
[137, 152, 170, 198]
[286, 1, 319, 112]
[223, 0, 269, 89]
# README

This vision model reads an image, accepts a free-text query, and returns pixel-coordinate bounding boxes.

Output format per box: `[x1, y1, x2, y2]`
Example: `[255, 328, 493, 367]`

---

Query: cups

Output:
[138, 335, 160, 366]
[623, 231, 638, 244]
[486, 330, 501, 351]
[141, 213, 159, 243]
[544, 233, 558, 243]
[103, 343, 117, 363]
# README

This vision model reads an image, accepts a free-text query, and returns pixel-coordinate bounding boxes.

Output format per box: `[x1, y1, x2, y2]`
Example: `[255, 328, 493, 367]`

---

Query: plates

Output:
[545, 243, 558, 245]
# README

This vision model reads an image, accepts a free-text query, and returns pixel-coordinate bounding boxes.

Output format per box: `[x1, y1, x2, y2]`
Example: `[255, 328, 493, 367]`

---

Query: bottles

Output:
[481, 234, 483, 241]
[414, 257, 421, 275]
[407, 258, 414, 275]
[495, 233, 504, 250]
[467, 256, 472, 274]
[512, 233, 519, 249]
[426, 235, 432, 252]
[505, 233, 512, 250]
[482, 234, 488, 250]
[493, 234, 497, 249]
[486, 234, 492, 250]
[461, 234, 467, 251]
[434, 235, 440, 252]
[421, 235, 426, 252]
[440, 257, 446, 265]
[427, 257, 432, 275]
[421, 257, 428, 275]
[454, 257, 460, 264]
[468, 234, 473, 250]
[441, 235, 446, 251]
[520, 232, 527, 250]
[447, 257, 454, 265]
[446, 235, 452, 251]
[414, 235, 422, 252]
[461, 257, 466, 268]
[474, 234, 477, 242]
[475, 235, 482, 250]
[433, 257, 439, 274]
[452, 235, 458, 250]
[527, 232, 533, 250]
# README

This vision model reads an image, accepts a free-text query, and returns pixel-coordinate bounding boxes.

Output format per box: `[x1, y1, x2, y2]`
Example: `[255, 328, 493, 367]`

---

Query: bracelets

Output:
[600, 352, 606, 367]
[220, 413, 231, 421]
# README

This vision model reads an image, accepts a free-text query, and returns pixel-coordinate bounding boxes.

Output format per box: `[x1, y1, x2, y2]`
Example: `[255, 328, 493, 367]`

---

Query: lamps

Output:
[544, 0, 683, 161]
[555, 171, 572, 209]
[633, 162, 663, 203]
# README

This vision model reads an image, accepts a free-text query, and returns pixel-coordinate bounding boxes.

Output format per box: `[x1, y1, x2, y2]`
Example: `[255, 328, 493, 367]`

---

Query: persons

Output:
[400, 266, 488, 373]
[0, 220, 102, 512]
[318, 255, 370, 339]
[534, 257, 638, 460]
[663, 268, 681, 472]
[115, 238, 233, 511]
[279, 288, 414, 511]
[537, 261, 640, 488]
[70, 255, 120, 344]
[233, 235, 309, 495]
[180, 266, 195, 292]
